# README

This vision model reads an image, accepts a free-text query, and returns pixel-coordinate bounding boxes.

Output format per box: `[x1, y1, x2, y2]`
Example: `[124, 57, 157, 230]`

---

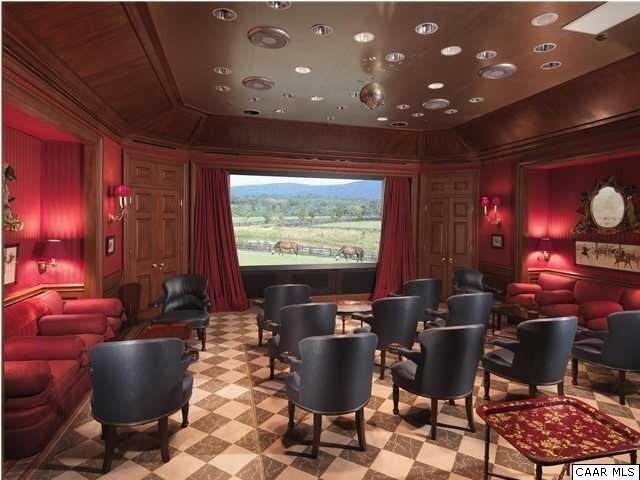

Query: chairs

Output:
[2, 263, 640, 479]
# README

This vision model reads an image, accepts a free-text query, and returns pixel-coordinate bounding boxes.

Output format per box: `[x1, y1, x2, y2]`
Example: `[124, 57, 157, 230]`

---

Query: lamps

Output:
[30, 235, 68, 277]
[535, 235, 555, 262]
[478, 193, 505, 227]
[105, 182, 135, 225]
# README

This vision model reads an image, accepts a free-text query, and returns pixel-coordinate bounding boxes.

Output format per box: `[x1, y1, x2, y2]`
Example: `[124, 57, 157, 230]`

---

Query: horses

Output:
[335, 245, 364, 263]
[271, 241, 298, 257]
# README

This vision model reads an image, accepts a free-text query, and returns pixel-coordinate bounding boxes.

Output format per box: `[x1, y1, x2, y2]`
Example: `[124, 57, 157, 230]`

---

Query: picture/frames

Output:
[570, 237, 640, 277]
[2, 240, 21, 289]
[488, 233, 506, 250]
[105, 234, 116, 257]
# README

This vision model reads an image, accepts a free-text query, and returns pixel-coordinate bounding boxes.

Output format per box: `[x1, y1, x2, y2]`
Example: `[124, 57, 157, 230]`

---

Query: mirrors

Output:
[569, 174, 639, 236]
